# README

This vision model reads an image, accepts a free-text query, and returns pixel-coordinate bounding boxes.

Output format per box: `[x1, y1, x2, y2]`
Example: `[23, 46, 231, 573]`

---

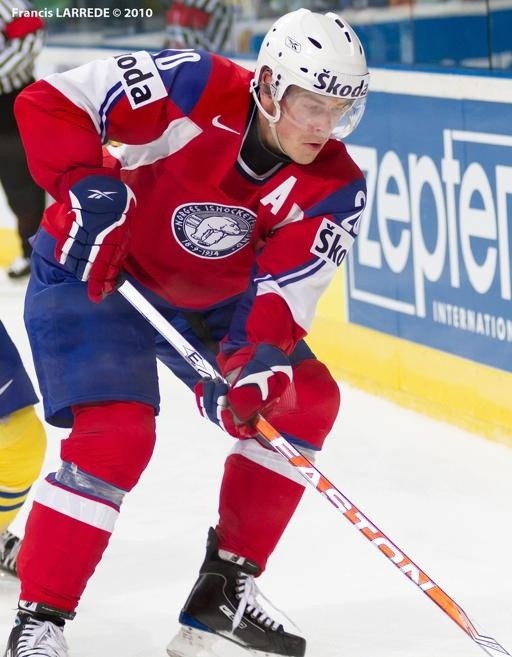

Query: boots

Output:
[176, 526, 307, 657]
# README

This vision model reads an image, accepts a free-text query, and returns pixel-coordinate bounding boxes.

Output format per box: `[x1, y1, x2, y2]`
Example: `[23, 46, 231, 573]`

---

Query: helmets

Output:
[248, 8, 373, 142]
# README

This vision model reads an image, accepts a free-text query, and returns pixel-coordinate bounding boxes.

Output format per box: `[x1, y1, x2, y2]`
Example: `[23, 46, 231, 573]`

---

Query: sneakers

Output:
[3, 597, 75, 657]
[0, 528, 24, 578]
[6, 254, 30, 280]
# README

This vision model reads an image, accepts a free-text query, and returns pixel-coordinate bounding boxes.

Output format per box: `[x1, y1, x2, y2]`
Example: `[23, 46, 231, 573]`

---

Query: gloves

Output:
[54, 173, 140, 305]
[194, 335, 298, 439]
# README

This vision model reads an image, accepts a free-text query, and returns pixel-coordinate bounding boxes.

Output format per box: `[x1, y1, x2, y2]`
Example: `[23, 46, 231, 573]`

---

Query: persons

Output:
[0, 0, 371, 657]
